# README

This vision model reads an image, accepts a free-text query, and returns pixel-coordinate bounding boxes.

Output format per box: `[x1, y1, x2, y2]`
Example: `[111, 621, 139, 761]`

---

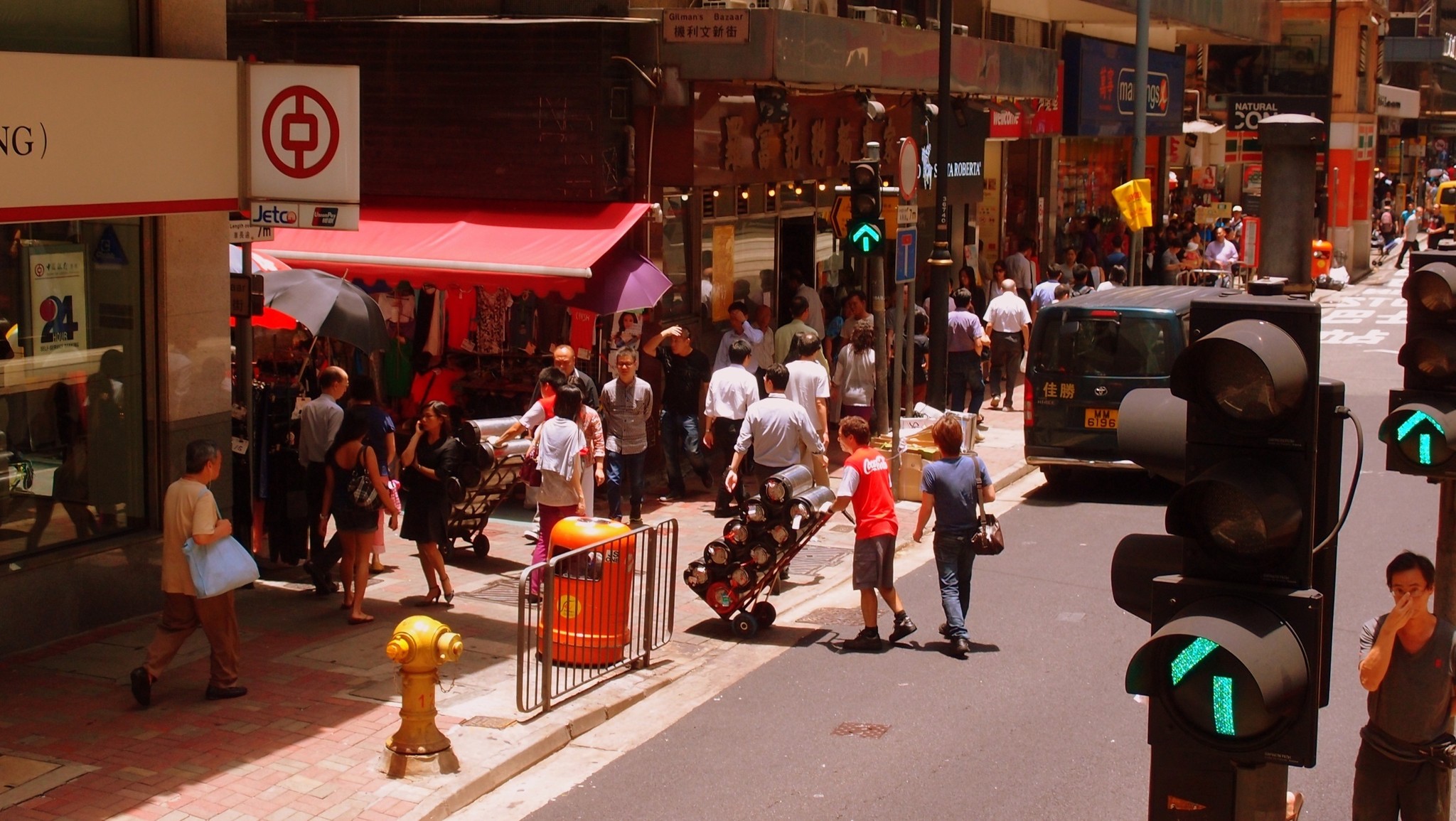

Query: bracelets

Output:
[319, 513, 328, 519]
[728, 465, 738, 471]
[705, 428, 713, 433]
[415, 464, 421, 469]
[827, 506, 835, 514]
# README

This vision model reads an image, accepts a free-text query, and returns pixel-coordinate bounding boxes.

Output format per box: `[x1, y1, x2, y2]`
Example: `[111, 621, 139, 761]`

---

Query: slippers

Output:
[342, 601, 350, 608]
[348, 615, 373, 624]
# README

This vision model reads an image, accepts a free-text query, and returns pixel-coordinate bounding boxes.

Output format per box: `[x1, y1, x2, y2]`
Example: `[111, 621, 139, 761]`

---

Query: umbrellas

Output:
[254, 268, 390, 382]
[567, 246, 674, 317]
[229, 242, 292, 275]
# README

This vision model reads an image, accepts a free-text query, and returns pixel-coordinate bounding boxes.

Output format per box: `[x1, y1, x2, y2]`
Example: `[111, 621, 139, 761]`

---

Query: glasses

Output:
[994, 268, 1005, 273]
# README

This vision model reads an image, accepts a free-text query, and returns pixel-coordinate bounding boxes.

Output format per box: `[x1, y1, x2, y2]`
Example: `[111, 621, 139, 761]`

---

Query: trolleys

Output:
[438, 452, 531, 560]
[683, 499, 855, 638]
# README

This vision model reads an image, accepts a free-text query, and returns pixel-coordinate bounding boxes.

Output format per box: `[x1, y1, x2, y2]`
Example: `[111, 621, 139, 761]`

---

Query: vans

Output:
[1427, 181, 1456, 229]
[1022, 285, 1246, 492]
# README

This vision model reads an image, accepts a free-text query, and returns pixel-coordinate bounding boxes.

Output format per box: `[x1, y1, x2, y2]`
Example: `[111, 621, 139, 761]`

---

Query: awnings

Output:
[233, 195, 663, 301]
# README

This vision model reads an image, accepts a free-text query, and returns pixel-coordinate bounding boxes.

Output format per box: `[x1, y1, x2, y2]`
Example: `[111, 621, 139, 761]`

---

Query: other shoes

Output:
[528, 593, 541, 603]
[206, 684, 247, 700]
[702, 470, 712, 488]
[990, 396, 1000, 408]
[660, 491, 682, 501]
[952, 638, 970, 653]
[1002, 407, 1014, 411]
[130, 667, 152, 708]
[939, 623, 950, 633]
[844, 630, 883, 653]
[304, 558, 337, 593]
[526, 529, 540, 542]
[630, 515, 641, 522]
[889, 617, 917, 642]
[714, 506, 739, 518]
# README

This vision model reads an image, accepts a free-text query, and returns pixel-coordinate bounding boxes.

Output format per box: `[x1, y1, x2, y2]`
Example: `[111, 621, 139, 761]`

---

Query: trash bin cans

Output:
[534, 515, 637, 669]
[1311, 239, 1333, 279]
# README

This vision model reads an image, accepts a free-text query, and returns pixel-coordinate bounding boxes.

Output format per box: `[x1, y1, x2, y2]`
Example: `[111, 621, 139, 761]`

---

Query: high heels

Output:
[415, 586, 441, 607]
[440, 574, 454, 602]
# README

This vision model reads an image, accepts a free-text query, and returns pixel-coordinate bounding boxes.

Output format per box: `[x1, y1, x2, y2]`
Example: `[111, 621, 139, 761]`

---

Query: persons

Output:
[1352, 549, 1456, 821]
[130, 439, 248, 711]
[826, 416, 917, 651]
[228, 168, 1244, 625]
[1201, 167, 1214, 190]
[1372, 143, 1456, 270]
[0, 317, 130, 558]
[913, 412, 996, 656]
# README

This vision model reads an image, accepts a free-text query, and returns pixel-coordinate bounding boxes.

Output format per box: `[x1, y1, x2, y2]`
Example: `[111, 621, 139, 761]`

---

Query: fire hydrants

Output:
[381, 614, 463, 776]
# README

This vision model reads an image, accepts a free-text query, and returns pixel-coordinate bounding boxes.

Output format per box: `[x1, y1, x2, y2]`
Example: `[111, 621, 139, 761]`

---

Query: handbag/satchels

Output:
[384, 480, 402, 514]
[971, 514, 1004, 556]
[348, 445, 384, 510]
[175, 483, 260, 599]
[520, 421, 546, 487]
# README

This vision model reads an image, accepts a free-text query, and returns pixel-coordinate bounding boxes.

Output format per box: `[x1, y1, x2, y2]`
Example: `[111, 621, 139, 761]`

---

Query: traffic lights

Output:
[1110, 290, 1321, 771]
[1378, 253, 1456, 483]
[848, 158, 886, 259]
